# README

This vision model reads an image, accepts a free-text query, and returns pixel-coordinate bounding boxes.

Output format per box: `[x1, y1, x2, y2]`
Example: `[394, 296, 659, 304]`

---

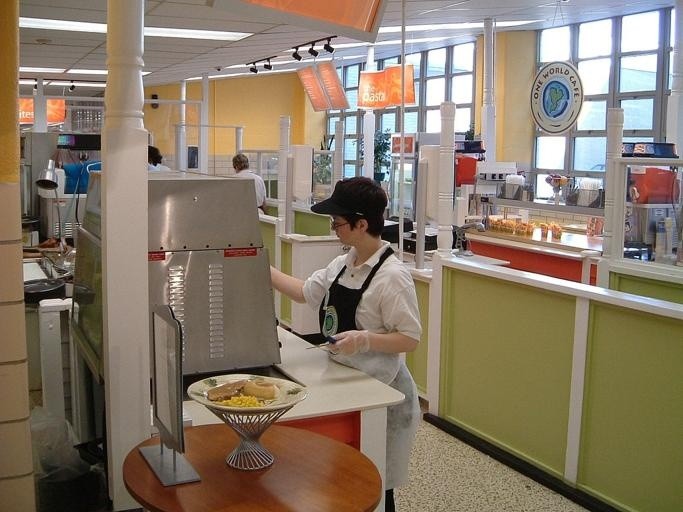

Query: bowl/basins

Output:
[501, 182, 520, 199]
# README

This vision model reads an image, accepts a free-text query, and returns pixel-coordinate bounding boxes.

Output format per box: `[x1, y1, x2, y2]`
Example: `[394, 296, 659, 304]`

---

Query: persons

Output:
[232, 154, 267, 215]
[271, 176, 421, 512]
[148, 146, 170, 171]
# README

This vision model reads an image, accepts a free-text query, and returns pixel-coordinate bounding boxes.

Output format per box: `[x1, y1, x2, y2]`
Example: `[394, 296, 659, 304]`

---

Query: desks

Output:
[123, 423, 383, 511]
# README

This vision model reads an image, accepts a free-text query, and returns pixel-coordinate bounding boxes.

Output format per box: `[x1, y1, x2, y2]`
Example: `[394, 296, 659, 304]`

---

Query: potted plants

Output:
[352, 129, 391, 181]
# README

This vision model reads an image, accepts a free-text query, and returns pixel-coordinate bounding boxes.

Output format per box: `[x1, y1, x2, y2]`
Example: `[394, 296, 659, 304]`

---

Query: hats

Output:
[307, 179, 387, 222]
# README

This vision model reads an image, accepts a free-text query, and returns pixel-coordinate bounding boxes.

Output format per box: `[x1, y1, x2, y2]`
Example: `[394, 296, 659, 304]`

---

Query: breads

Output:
[241, 380, 280, 399]
[206, 379, 248, 401]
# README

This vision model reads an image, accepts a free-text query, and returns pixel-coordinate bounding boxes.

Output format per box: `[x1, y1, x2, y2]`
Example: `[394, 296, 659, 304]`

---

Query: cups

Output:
[488, 224, 561, 240]
[517, 190, 534, 202]
[654, 215, 674, 263]
[564, 188, 580, 206]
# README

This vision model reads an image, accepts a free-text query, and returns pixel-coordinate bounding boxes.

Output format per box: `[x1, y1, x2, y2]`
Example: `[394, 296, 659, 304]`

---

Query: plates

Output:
[22, 278, 67, 293]
[186, 373, 311, 416]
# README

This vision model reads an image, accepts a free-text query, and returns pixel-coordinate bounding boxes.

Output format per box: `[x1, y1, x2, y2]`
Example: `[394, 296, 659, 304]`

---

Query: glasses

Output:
[328, 216, 356, 230]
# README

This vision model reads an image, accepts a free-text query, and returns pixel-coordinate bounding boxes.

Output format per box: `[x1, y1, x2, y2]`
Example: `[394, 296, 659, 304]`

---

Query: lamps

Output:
[35, 160, 62, 245]
[292, 35, 338, 62]
[245, 55, 279, 73]
[20, 78, 89, 92]
